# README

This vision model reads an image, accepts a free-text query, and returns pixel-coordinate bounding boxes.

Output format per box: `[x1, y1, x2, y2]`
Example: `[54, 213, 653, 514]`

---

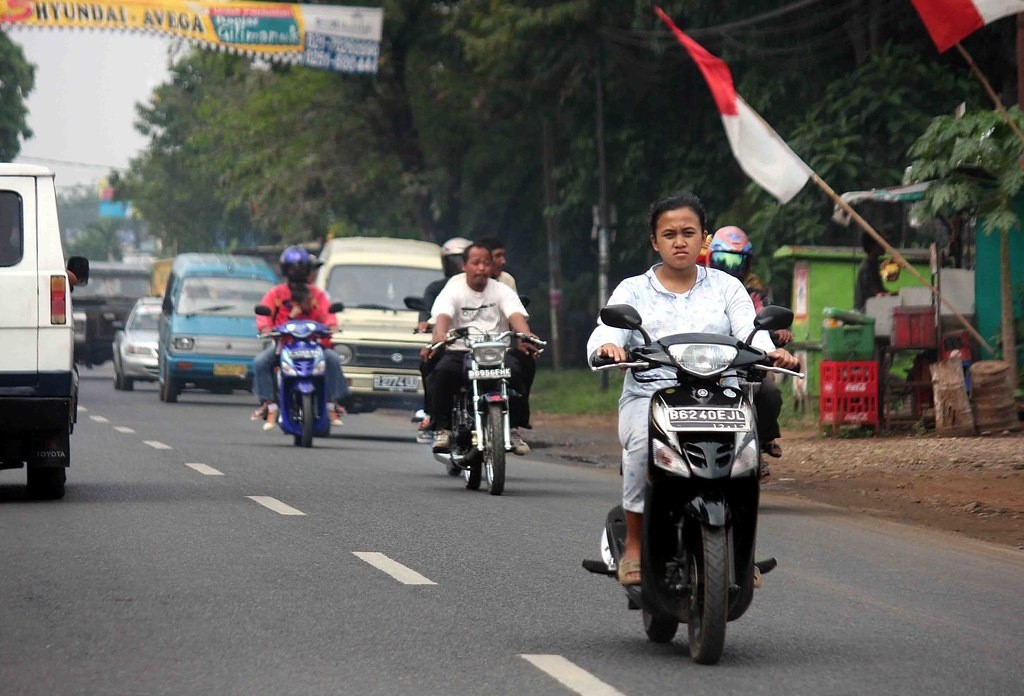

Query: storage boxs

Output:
[816, 267, 976, 435]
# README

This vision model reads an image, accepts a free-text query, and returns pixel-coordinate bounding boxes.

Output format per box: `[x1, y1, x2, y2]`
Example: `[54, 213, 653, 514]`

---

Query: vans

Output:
[155, 253, 284, 402]
[0, 162, 91, 502]
[307, 234, 448, 412]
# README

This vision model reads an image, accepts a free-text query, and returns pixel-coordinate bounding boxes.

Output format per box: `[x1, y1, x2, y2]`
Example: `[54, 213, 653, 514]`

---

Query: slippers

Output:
[619, 554, 641, 585]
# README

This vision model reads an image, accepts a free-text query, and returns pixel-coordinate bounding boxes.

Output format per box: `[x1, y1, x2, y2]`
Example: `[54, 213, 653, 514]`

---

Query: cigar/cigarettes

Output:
[534, 348, 544, 355]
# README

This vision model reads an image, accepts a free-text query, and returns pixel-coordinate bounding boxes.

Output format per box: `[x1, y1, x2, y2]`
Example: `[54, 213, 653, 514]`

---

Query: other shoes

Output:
[416, 428, 433, 443]
[754, 565, 761, 588]
[764, 443, 782, 458]
[758, 461, 771, 484]
[329, 408, 343, 426]
[250, 404, 266, 420]
[262, 406, 279, 432]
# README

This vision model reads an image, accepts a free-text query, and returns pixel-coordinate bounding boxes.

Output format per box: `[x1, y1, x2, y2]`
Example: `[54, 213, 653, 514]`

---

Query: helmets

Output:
[706, 225, 754, 282]
[439, 236, 474, 275]
[278, 246, 325, 281]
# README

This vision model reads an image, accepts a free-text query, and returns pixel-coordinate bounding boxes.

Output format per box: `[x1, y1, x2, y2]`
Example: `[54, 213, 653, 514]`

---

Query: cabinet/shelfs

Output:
[884, 343, 940, 435]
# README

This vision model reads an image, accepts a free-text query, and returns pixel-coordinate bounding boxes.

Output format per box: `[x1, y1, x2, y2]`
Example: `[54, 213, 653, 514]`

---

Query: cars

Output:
[112, 297, 235, 395]
[72, 263, 156, 376]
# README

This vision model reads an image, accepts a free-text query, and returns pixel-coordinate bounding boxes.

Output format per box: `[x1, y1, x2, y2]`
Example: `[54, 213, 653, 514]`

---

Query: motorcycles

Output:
[428, 325, 547, 496]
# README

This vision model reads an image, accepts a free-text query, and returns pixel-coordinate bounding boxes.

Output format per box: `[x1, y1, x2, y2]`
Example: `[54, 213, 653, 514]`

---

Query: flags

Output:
[653, 4, 813, 205]
[911, 0, 1024, 53]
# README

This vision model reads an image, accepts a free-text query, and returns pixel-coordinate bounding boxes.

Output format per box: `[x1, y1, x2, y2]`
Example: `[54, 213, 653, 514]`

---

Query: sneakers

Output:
[509, 428, 531, 456]
[432, 429, 452, 454]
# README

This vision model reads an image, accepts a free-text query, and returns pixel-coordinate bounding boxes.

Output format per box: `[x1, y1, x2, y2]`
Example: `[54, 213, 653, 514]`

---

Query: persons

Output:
[416, 237, 540, 456]
[0, 203, 77, 285]
[250, 245, 350, 432]
[587, 195, 799, 587]
[697, 225, 793, 458]
[854, 230, 898, 311]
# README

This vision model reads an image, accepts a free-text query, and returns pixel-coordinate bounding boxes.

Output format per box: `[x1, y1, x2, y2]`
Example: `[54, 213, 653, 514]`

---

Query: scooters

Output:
[253, 301, 346, 446]
[582, 303, 806, 665]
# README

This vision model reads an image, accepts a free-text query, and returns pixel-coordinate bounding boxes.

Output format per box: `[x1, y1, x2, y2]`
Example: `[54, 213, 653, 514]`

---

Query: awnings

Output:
[832, 178, 977, 226]
